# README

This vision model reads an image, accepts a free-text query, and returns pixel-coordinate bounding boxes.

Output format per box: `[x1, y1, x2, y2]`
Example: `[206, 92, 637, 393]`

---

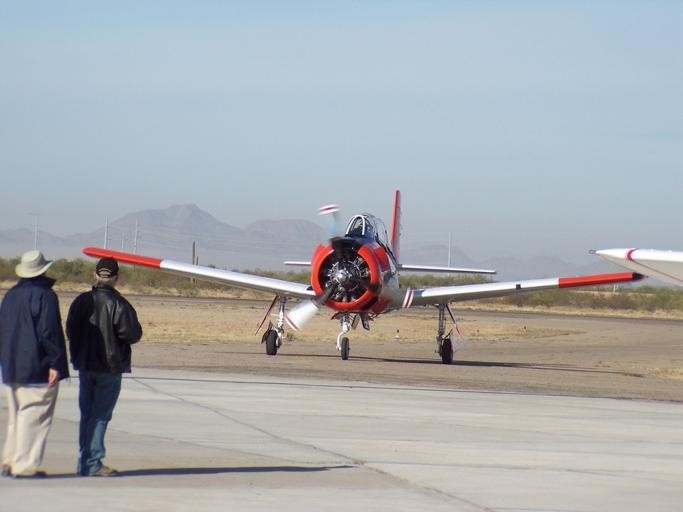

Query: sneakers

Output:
[83, 464, 116, 476]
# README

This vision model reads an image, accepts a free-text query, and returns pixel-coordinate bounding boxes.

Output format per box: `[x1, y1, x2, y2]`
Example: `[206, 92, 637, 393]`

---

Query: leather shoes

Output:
[1, 465, 10, 476]
[12, 471, 45, 478]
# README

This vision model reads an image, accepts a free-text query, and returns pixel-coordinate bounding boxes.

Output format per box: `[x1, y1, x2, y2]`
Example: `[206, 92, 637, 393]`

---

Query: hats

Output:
[95, 257, 118, 278]
[14, 250, 53, 278]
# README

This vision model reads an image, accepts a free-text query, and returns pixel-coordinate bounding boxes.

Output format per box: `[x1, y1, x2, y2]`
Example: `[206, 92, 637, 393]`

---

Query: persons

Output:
[65, 256, 144, 477]
[0, 250, 70, 478]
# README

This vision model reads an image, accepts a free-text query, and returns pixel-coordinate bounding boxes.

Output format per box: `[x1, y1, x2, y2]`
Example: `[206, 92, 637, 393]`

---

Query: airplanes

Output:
[585, 240, 683, 289]
[75, 184, 650, 369]
